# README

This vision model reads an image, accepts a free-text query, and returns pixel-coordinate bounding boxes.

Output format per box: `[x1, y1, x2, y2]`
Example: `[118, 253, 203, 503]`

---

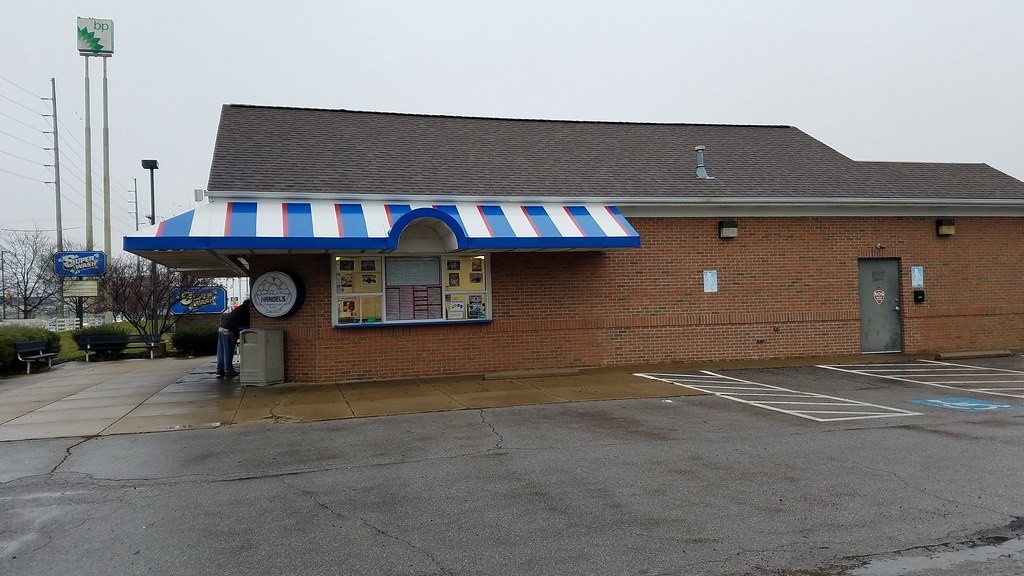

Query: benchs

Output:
[13, 341, 58, 375]
[77, 334, 160, 363]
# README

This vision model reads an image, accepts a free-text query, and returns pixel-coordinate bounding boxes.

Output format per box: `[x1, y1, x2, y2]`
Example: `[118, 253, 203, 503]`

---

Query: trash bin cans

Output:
[239, 326, 286, 386]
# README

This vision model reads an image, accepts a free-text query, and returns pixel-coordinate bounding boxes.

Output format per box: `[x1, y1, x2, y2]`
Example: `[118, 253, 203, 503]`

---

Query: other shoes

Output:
[216, 368, 226, 375]
[224, 368, 238, 376]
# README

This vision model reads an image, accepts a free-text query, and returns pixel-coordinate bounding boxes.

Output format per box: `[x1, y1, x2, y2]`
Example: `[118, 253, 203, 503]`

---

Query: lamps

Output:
[719, 220, 738, 239]
[936, 219, 955, 237]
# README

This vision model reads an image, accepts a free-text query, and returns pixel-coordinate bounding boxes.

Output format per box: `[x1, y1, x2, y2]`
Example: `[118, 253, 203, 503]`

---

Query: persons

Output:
[216, 300, 251, 378]
